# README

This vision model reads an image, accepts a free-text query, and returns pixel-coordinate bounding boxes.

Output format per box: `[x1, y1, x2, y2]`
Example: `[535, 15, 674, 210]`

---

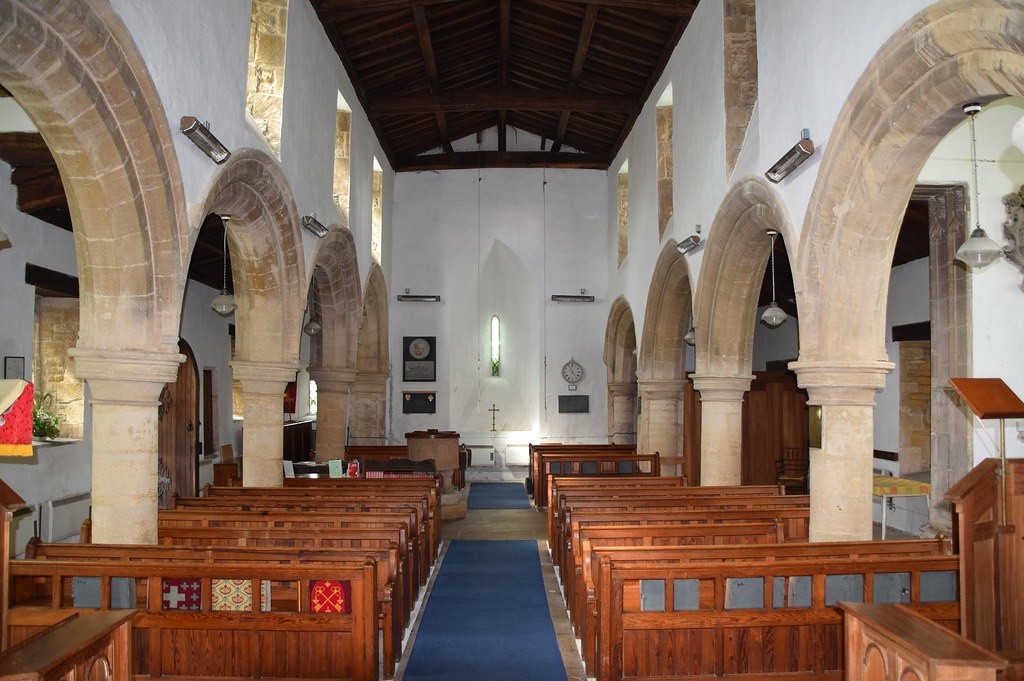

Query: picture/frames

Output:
[4, 355, 26, 381]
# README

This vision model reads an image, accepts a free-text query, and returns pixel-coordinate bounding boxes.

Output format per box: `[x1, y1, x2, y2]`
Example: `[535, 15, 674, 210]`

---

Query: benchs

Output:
[525, 443, 958, 680]
[8, 474, 442, 681]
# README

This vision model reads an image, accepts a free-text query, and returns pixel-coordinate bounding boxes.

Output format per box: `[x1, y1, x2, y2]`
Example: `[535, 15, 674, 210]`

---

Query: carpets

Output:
[466, 483, 532, 510]
[402, 539, 569, 680]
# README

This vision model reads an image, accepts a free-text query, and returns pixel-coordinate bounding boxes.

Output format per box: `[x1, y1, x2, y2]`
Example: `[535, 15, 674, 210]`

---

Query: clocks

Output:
[562, 362, 584, 384]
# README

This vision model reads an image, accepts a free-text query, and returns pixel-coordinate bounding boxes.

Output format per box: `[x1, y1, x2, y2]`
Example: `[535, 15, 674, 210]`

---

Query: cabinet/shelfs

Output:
[839, 607, 1006, 681]
[958, 466, 1023, 681]
[0, 608, 139, 680]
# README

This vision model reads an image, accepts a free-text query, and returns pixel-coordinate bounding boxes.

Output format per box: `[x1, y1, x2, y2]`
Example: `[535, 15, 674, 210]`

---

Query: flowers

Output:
[29, 399, 63, 443]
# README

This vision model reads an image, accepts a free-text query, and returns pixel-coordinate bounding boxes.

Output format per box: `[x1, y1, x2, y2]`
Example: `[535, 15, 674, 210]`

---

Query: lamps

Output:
[954, 103, 1003, 268]
[683, 327, 697, 346]
[551, 289, 595, 303]
[765, 128, 814, 185]
[302, 273, 323, 337]
[210, 214, 238, 317]
[303, 213, 330, 239]
[397, 288, 440, 303]
[180, 117, 232, 165]
[675, 226, 702, 258]
[759, 228, 788, 326]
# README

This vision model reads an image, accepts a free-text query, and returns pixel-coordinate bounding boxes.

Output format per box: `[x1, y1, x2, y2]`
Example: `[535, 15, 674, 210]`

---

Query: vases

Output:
[32, 436, 47, 442]
